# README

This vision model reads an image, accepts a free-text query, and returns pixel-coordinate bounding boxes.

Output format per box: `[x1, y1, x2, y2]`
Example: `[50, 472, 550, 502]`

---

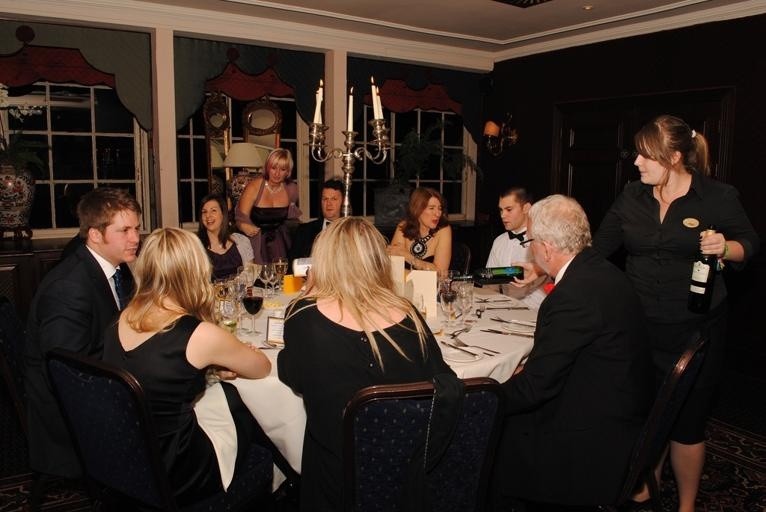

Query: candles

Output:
[312, 75, 383, 129]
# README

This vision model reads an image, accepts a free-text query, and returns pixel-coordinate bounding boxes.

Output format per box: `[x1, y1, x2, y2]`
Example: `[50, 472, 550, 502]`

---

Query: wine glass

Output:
[213, 258, 287, 318]
[242, 289, 263, 337]
[413, 261, 474, 333]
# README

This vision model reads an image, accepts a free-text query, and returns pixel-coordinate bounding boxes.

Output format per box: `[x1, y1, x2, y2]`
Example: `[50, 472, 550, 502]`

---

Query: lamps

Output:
[483, 111, 520, 158]
[223, 141, 264, 207]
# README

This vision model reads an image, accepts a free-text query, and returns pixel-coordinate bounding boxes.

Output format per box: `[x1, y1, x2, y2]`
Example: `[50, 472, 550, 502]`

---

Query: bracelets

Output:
[721, 239, 729, 259]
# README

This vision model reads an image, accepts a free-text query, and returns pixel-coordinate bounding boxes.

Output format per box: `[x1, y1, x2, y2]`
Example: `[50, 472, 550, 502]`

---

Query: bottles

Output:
[449, 266, 526, 286]
[686, 224, 720, 314]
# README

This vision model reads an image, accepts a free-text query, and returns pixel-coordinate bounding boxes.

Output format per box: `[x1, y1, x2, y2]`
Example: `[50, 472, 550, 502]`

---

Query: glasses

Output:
[520, 239, 534, 249]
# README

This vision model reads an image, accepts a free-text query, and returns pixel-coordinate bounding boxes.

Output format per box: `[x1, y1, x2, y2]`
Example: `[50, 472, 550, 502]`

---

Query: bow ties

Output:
[507, 230, 527, 242]
[543, 281, 555, 295]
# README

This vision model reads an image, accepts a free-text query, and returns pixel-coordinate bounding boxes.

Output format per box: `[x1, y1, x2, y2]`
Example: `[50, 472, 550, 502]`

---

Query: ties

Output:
[113, 270, 127, 313]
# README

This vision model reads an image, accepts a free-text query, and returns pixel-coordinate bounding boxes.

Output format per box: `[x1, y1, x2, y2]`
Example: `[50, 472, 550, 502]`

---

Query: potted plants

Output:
[0, 118, 58, 229]
[370, 117, 486, 236]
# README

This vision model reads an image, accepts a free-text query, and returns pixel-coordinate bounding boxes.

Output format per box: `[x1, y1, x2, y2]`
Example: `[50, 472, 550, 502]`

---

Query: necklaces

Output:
[264, 180, 285, 193]
[409, 231, 435, 260]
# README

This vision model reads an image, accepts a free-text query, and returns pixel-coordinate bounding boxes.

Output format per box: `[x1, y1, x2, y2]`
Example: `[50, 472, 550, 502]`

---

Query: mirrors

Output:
[204, 92, 232, 212]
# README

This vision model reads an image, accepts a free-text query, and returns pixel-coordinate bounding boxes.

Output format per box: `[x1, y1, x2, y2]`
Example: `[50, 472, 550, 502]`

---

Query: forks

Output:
[455, 337, 501, 354]
[451, 328, 472, 338]
[480, 305, 486, 312]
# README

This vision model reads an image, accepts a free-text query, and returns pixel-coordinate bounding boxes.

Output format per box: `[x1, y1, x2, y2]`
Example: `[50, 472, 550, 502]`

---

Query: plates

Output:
[445, 347, 482, 363]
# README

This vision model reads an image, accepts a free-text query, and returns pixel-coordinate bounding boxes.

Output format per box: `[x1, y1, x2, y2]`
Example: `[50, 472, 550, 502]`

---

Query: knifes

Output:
[440, 339, 477, 359]
[480, 328, 533, 340]
[475, 300, 511, 303]
[489, 317, 535, 327]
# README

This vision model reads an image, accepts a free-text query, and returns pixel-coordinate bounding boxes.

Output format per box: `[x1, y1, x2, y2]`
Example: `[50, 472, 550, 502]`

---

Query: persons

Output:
[481, 193, 650, 510]
[288, 178, 349, 272]
[22, 186, 142, 487]
[386, 188, 453, 283]
[479, 184, 555, 315]
[587, 114, 757, 512]
[276, 216, 457, 511]
[100, 227, 271, 507]
[194, 196, 254, 281]
[236, 148, 303, 266]
